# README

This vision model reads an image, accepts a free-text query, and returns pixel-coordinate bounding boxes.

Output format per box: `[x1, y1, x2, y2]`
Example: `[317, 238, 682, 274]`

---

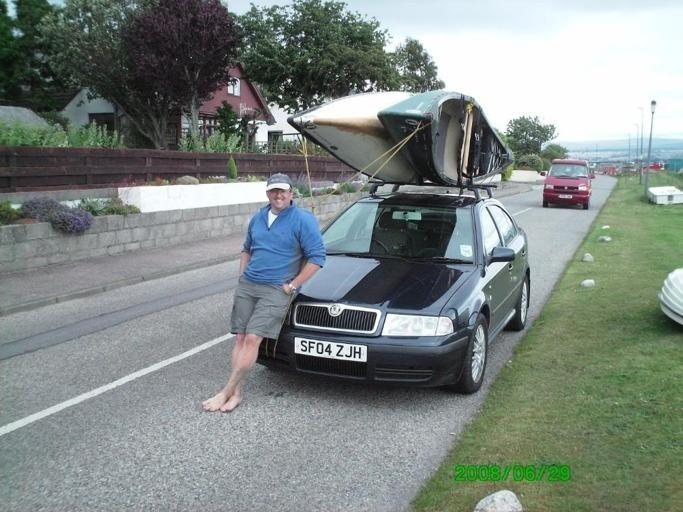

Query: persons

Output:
[202, 173, 326, 412]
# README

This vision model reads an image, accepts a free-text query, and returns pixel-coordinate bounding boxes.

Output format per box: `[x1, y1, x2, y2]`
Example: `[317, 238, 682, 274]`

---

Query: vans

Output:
[540, 158, 595, 210]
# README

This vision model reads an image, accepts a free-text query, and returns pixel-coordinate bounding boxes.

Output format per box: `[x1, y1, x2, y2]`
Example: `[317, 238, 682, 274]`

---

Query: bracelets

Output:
[289, 284, 297, 292]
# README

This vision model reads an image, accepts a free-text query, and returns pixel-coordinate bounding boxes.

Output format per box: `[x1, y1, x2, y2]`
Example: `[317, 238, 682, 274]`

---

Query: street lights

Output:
[644, 101, 656, 196]
[626, 105, 644, 184]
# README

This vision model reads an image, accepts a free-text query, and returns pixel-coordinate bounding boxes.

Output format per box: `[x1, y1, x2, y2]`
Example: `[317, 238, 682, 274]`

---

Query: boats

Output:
[286, 90, 515, 186]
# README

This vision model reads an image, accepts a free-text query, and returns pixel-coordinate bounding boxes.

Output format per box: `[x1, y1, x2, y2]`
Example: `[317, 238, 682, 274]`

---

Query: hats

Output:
[265, 173, 293, 191]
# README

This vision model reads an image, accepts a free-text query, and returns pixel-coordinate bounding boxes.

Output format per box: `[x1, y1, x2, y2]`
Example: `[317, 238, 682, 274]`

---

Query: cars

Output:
[255, 180, 531, 395]
